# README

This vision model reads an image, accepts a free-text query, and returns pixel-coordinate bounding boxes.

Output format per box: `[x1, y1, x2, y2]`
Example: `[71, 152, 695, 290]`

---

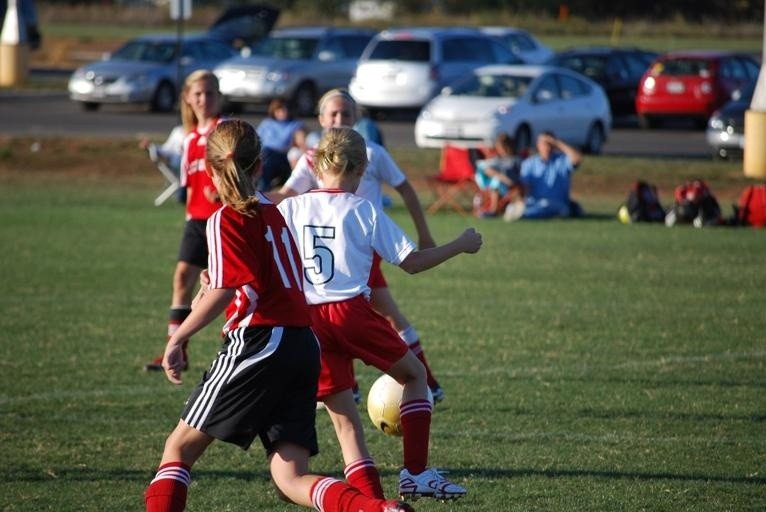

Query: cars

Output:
[540, 46, 662, 115]
[66, 8, 280, 112]
[409, 67, 614, 154]
[482, 26, 559, 64]
[634, 49, 762, 129]
[701, 65, 761, 161]
[212, 26, 376, 118]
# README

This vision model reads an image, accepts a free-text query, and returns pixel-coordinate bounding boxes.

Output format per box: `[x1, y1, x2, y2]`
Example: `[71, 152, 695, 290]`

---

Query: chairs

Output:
[425, 143, 490, 218]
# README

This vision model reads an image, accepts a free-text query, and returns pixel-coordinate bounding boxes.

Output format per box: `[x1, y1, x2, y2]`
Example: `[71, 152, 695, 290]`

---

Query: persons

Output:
[251, 96, 309, 194]
[274, 125, 483, 503]
[500, 130, 583, 225]
[144, 70, 228, 372]
[285, 125, 310, 169]
[469, 133, 524, 219]
[263, 89, 445, 410]
[132, 121, 189, 204]
[143, 118, 416, 512]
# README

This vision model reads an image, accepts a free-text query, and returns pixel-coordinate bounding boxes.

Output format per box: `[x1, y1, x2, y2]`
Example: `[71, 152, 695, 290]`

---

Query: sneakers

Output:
[384, 501, 416, 511]
[315, 389, 361, 410]
[143, 355, 191, 372]
[398, 469, 466, 502]
[502, 198, 527, 223]
[430, 382, 445, 405]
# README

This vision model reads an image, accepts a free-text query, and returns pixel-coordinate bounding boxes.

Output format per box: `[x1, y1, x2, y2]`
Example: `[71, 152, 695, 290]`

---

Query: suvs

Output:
[347, 26, 529, 118]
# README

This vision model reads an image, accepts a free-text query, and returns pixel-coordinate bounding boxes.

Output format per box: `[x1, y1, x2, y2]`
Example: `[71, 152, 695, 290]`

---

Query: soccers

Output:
[368, 374, 433, 434]
